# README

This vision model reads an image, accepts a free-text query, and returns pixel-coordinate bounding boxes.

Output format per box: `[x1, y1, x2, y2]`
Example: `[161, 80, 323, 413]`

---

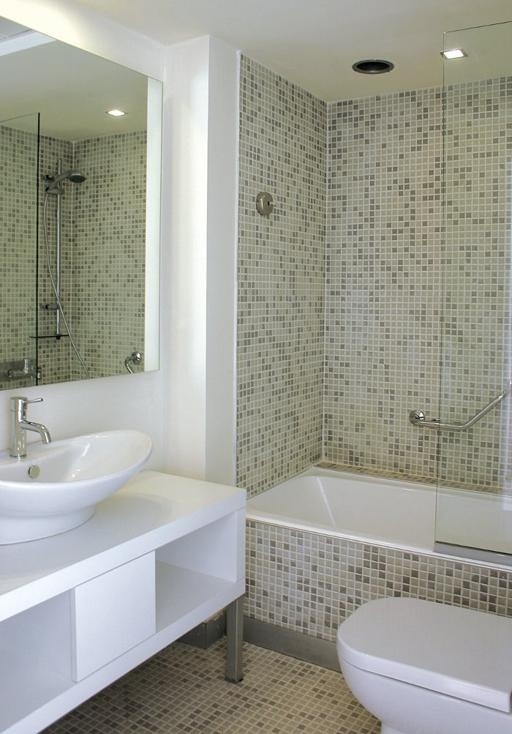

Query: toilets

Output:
[336, 597, 512, 734]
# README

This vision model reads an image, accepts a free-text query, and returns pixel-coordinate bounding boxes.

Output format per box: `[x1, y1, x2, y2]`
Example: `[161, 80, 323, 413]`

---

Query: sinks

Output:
[0, 428, 152, 544]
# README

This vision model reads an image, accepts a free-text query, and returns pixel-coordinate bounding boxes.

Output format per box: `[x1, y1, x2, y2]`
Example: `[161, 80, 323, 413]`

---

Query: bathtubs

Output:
[246, 470, 512, 572]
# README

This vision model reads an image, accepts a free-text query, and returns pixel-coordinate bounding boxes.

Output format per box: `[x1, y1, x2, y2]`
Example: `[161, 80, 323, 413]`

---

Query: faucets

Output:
[10, 396, 52, 457]
[22, 358, 41, 380]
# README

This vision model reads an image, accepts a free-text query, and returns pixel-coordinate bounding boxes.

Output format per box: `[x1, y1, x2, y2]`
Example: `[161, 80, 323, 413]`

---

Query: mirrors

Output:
[0, 15, 165, 395]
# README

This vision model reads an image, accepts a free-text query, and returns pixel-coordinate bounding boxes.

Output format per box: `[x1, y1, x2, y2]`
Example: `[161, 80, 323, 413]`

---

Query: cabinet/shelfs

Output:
[1, 470, 248, 734]
